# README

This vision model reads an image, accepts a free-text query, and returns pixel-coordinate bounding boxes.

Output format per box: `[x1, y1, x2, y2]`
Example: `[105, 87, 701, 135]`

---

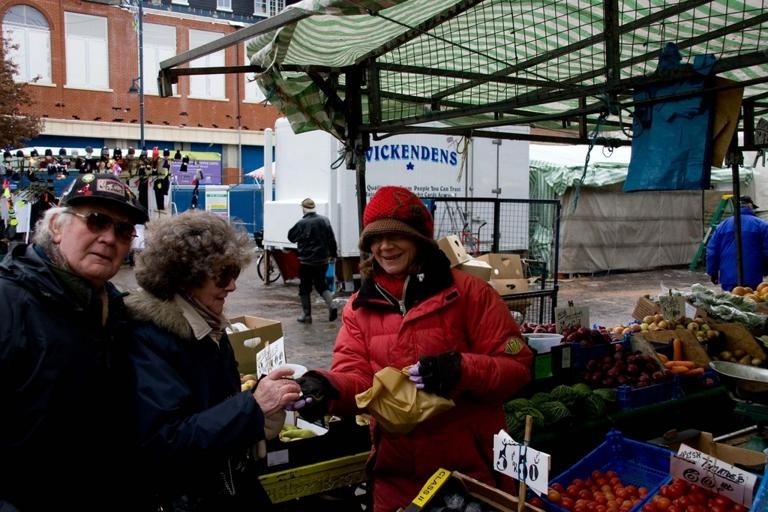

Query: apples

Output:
[732, 280, 768, 305]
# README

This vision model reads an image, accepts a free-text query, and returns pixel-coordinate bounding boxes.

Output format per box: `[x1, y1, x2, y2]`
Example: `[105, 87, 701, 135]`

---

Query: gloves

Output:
[408, 351, 460, 397]
[711, 275, 718, 284]
[285, 373, 328, 415]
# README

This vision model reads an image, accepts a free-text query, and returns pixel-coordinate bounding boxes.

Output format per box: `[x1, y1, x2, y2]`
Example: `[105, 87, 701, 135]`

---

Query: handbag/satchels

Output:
[355, 365, 457, 440]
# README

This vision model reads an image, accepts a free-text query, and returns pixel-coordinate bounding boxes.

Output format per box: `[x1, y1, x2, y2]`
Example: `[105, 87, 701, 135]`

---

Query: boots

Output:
[321, 290, 337, 321]
[297, 293, 311, 323]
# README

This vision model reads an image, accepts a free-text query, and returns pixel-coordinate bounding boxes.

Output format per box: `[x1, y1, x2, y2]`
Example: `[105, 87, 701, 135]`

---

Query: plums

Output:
[584, 343, 660, 390]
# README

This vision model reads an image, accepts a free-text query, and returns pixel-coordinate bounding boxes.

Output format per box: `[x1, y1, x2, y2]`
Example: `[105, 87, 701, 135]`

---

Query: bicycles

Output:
[252, 228, 284, 286]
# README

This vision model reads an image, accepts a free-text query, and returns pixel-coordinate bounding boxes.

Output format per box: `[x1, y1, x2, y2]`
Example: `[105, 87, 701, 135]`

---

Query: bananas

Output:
[689, 315, 721, 344]
[656, 338, 702, 376]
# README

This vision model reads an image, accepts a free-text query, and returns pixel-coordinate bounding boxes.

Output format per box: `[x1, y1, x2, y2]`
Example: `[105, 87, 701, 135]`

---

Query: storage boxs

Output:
[521, 330, 728, 409]
[401, 469, 542, 512]
[259, 420, 370, 504]
[486, 279, 529, 297]
[455, 250, 492, 279]
[477, 252, 527, 278]
[527, 429, 768, 512]
[438, 233, 469, 262]
[222, 314, 284, 377]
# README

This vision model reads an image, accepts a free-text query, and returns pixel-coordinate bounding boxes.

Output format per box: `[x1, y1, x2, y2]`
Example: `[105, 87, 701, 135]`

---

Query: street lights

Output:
[126, 0, 149, 155]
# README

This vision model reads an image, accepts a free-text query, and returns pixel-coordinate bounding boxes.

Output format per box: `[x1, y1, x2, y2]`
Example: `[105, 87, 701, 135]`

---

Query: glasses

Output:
[61, 210, 138, 245]
[209, 264, 240, 289]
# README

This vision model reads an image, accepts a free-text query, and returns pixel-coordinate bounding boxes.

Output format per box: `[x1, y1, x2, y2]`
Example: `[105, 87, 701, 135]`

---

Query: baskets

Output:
[526, 429, 768, 511]
[559, 295, 721, 411]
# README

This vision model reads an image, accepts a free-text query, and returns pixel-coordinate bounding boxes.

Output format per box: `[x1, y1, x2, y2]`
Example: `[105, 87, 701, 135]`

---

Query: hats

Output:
[59, 173, 149, 224]
[359, 186, 439, 253]
[2, 145, 207, 185]
[740, 194, 759, 209]
[302, 198, 315, 208]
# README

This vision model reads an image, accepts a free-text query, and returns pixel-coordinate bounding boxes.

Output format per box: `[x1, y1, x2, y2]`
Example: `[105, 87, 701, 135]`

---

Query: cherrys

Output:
[644, 478, 747, 512]
[547, 468, 648, 512]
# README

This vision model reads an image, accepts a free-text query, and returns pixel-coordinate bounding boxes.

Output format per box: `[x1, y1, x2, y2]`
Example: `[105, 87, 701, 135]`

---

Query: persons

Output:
[99, 209, 302, 511]
[706, 195, 768, 292]
[0, 172, 150, 512]
[283, 185, 536, 512]
[287, 197, 338, 323]
